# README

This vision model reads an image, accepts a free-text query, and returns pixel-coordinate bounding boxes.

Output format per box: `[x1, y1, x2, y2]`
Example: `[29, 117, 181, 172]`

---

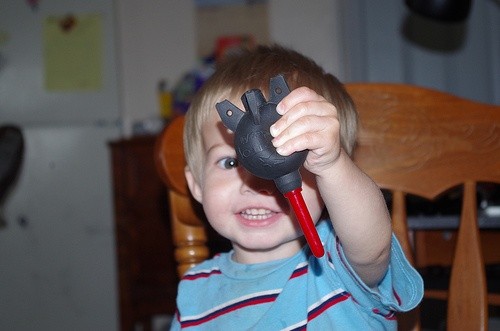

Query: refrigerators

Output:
[0, 0, 125, 331]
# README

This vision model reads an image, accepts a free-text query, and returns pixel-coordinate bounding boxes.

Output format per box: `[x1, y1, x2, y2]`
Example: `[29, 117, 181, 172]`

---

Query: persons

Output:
[164, 43, 426, 331]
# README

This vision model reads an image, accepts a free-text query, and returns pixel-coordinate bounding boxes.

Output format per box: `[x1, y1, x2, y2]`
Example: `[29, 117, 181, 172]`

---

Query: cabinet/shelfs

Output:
[107, 132, 216, 331]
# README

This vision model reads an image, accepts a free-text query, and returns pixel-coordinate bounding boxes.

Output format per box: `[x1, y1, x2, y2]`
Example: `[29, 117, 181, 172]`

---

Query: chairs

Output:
[155, 79, 498, 331]
